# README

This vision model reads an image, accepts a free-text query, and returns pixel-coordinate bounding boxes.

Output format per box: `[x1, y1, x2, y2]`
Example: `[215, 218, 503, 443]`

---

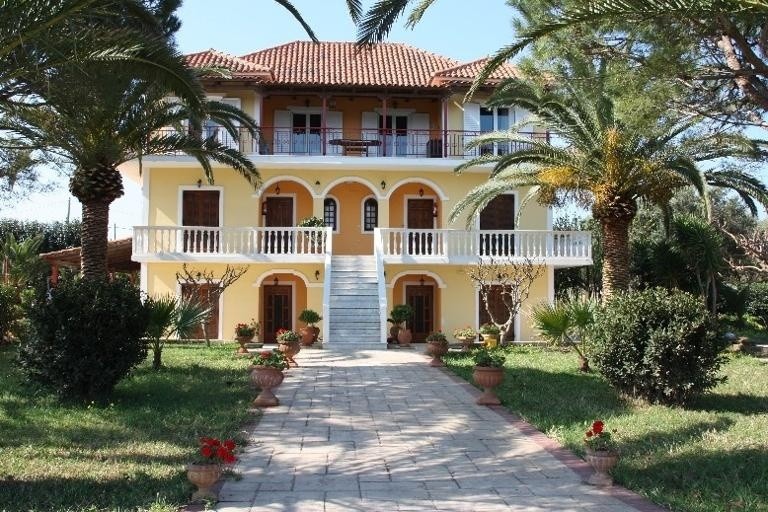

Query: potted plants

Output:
[296, 308, 323, 346]
[387, 317, 405, 344]
[309, 317, 322, 344]
[424, 330, 451, 370]
[475, 321, 500, 343]
[294, 213, 328, 253]
[452, 325, 477, 354]
[389, 303, 417, 347]
[470, 346, 510, 407]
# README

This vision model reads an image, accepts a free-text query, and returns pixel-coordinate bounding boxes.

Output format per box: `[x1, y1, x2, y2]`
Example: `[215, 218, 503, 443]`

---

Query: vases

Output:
[278, 341, 301, 368]
[186, 461, 219, 503]
[234, 336, 251, 355]
[249, 364, 283, 407]
[583, 446, 620, 486]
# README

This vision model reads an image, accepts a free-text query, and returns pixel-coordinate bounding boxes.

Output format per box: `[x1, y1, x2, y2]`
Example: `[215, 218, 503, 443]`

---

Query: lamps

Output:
[261, 200, 269, 216]
[419, 276, 425, 286]
[431, 203, 438, 217]
[380, 179, 386, 190]
[418, 188, 425, 198]
[274, 181, 281, 195]
[272, 276, 279, 286]
[314, 270, 321, 281]
[195, 177, 204, 191]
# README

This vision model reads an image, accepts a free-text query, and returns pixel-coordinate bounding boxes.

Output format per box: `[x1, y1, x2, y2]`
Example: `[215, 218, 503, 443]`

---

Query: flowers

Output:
[249, 347, 289, 369]
[581, 418, 618, 451]
[276, 327, 301, 343]
[190, 430, 240, 464]
[233, 323, 255, 338]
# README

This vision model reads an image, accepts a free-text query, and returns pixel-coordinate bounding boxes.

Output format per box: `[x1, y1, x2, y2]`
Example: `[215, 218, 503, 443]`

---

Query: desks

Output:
[327, 139, 382, 158]
